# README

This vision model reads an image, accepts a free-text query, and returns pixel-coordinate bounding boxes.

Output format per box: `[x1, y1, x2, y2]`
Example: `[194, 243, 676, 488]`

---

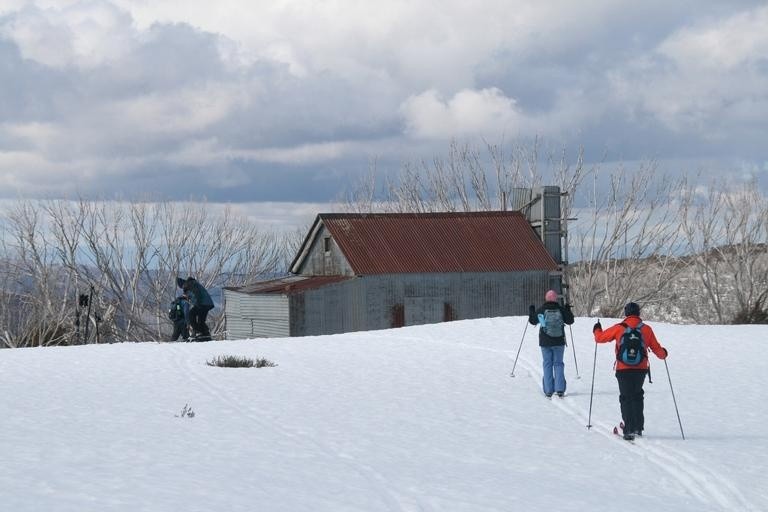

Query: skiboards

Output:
[614, 423, 643, 446]
[545, 391, 566, 402]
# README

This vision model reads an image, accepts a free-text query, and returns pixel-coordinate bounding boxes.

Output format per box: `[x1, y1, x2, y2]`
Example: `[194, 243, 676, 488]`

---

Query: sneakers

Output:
[545, 391, 564, 398]
[622, 428, 643, 441]
[189, 332, 213, 341]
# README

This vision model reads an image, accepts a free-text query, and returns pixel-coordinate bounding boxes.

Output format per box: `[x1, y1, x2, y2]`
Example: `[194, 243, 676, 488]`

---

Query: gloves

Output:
[593, 322, 602, 333]
[662, 347, 668, 358]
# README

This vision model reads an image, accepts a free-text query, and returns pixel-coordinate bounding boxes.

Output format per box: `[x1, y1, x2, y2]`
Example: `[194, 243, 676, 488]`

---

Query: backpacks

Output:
[537, 309, 566, 339]
[615, 322, 650, 366]
[168, 299, 184, 322]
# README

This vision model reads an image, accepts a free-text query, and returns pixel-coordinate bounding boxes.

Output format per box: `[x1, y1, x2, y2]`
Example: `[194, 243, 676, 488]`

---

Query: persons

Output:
[176, 276, 214, 342]
[170, 288, 193, 342]
[594, 302, 669, 440]
[528, 290, 574, 396]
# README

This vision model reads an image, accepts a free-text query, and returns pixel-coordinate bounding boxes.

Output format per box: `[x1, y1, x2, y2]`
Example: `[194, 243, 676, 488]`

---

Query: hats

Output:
[177, 277, 186, 289]
[545, 289, 557, 302]
[624, 302, 640, 318]
